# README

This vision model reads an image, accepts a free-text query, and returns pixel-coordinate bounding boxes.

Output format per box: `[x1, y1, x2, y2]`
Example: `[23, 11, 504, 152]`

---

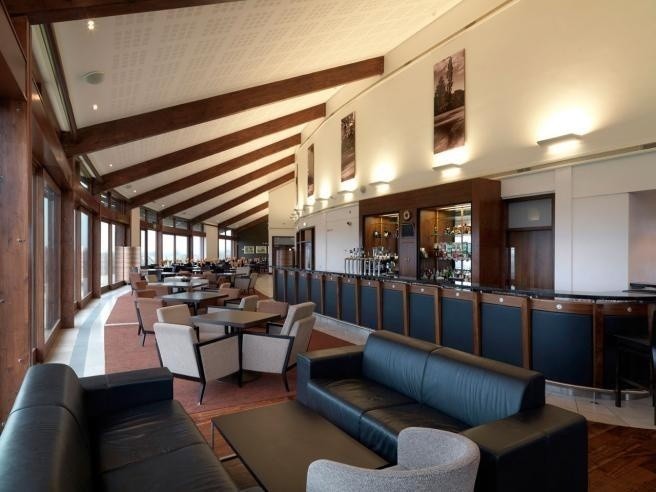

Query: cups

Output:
[342, 245, 398, 280]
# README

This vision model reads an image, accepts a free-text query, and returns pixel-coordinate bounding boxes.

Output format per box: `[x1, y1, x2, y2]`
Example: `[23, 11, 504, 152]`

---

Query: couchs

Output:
[1, 363, 240, 491]
[296, 329, 589, 491]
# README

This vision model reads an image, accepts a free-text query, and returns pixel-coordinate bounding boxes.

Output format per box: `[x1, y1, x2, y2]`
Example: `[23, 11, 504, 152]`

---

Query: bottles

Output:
[429, 222, 473, 287]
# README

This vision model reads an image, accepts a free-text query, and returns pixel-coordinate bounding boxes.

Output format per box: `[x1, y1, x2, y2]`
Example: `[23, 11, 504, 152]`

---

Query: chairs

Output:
[306, 426, 480, 492]
[128, 264, 315, 404]
